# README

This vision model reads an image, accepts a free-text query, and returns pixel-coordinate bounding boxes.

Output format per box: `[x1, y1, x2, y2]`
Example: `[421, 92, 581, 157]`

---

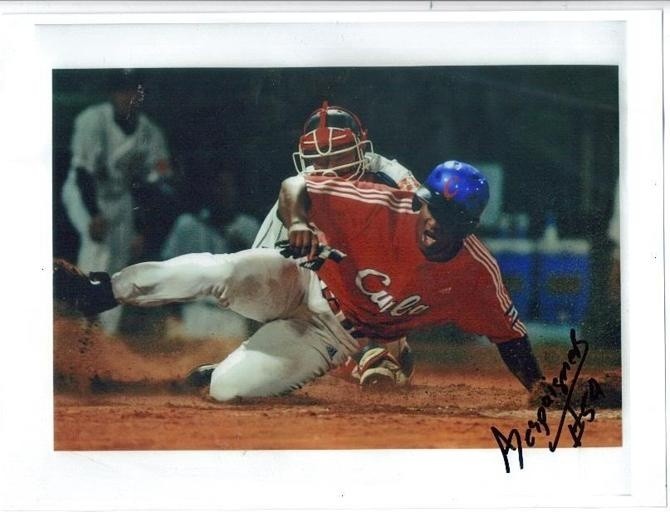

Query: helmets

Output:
[412, 159, 489, 240]
[291, 99, 376, 184]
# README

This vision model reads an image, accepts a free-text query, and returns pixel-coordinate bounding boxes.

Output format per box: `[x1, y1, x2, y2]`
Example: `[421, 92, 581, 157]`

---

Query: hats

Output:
[111, 69, 142, 90]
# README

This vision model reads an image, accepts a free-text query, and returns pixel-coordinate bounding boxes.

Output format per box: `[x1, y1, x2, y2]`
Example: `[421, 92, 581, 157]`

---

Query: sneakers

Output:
[53, 260, 117, 319]
[335, 336, 418, 391]
[186, 363, 217, 387]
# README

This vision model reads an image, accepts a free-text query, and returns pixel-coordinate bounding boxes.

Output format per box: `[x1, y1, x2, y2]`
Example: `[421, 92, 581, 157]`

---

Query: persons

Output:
[245, 100, 428, 390]
[601, 175, 620, 317]
[64, 195, 182, 337]
[54, 159, 566, 411]
[60, 69, 178, 341]
[155, 164, 261, 341]
